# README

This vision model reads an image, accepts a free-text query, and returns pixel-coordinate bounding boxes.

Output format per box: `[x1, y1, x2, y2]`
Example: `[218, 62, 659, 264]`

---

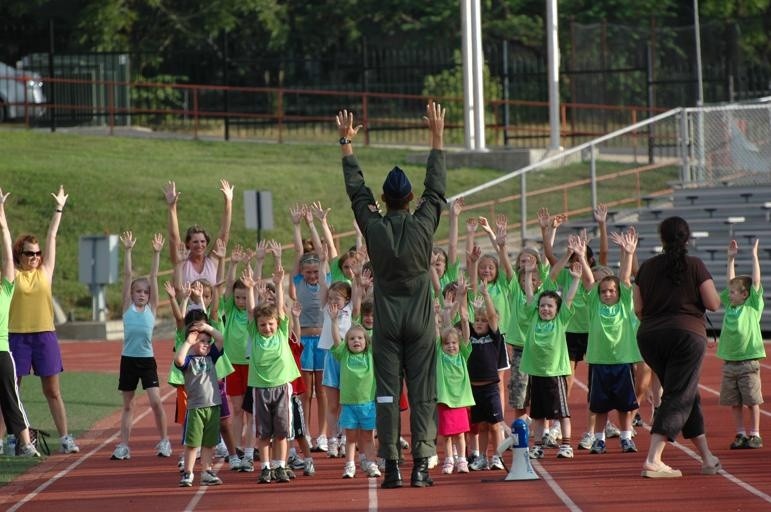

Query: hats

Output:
[384, 167, 411, 197]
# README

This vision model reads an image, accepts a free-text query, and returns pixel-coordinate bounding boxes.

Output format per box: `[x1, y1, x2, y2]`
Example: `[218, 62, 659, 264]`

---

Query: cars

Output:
[0, 61, 47, 122]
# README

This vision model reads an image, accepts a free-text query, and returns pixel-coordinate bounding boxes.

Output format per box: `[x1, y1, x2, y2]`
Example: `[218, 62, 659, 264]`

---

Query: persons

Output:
[332, 100, 449, 488]
[9, 184, 80, 455]
[108, 230, 171, 460]
[160, 196, 410, 488]
[160, 177, 239, 292]
[633, 215, 724, 477]
[714, 238, 766, 449]
[428, 195, 663, 473]
[0, 188, 42, 458]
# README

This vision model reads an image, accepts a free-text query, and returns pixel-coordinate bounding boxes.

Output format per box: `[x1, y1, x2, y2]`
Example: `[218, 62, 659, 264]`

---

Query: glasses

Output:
[22, 251, 42, 257]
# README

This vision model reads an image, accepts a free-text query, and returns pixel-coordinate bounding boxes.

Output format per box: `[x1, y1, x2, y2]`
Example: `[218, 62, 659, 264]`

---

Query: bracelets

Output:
[55, 208, 64, 213]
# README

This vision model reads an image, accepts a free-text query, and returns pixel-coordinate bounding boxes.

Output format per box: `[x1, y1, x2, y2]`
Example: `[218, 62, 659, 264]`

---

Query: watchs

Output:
[339, 137, 354, 144]
